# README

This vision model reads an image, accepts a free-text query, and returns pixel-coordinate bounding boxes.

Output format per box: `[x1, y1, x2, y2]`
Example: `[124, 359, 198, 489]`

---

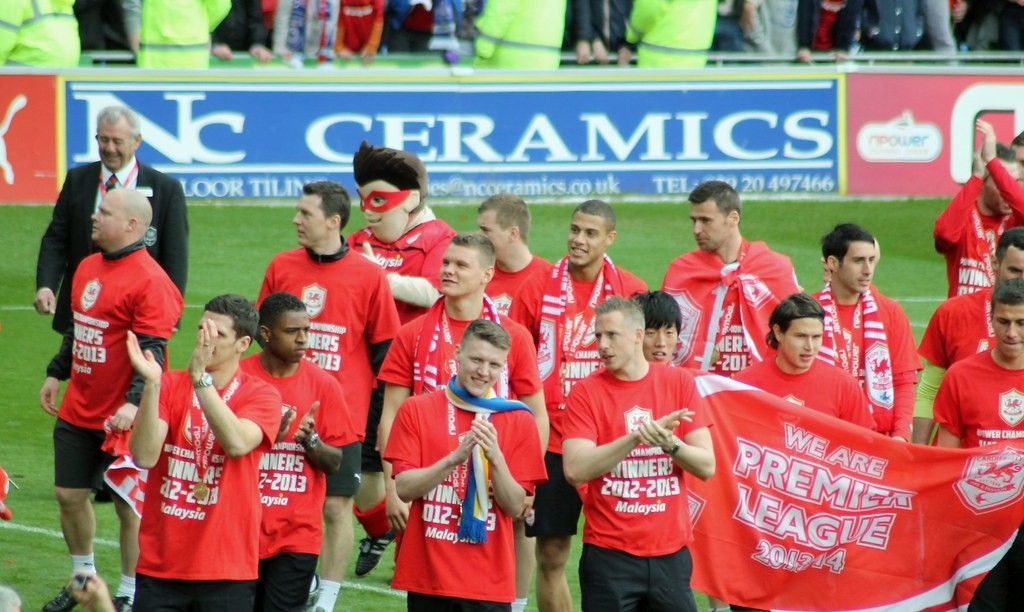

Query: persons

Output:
[0, 0, 1023, 70]
[240, 292, 360, 612]
[631, 180, 805, 612]
[811, 223, 924, 442]
[126, 294, 282, 612]
[562, 291, 716, 612]
[729, 293, 877, 612]
[40, 188, 184, 612]
[253, 181, 401, 612]
[478, 194, 649, 612]
[34, 105, 190, 504]
[383, 319, 549, 612]
[345, 141, 456, 576]
[378, 234, 550, 531]
[0, 569, 118, 612]
[912, 119, 1024, 446]
[933, 278, 1024, 612]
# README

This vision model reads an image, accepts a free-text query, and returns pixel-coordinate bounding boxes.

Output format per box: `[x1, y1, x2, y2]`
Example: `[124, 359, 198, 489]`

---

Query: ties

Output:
[106, 175, 118, 193]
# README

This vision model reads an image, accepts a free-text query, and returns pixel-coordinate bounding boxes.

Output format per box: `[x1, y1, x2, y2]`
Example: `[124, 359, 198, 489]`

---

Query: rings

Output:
[204, 343, 209, 346]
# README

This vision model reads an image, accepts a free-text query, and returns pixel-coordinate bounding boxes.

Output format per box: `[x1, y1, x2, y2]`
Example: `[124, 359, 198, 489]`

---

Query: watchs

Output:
[303, 430, 318, 450]
[662, 436, 681, 454]
[193, 373, 213, 388]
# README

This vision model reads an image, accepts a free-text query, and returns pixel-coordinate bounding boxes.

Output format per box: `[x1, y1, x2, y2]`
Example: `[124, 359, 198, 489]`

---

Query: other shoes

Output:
[302, 574, 320, 612]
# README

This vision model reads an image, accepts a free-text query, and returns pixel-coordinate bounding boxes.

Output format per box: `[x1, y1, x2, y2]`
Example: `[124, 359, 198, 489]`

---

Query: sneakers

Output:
[355, 527, 396, 575]
[42, 576, 91, 612]
[111, 596, 133, 612]
